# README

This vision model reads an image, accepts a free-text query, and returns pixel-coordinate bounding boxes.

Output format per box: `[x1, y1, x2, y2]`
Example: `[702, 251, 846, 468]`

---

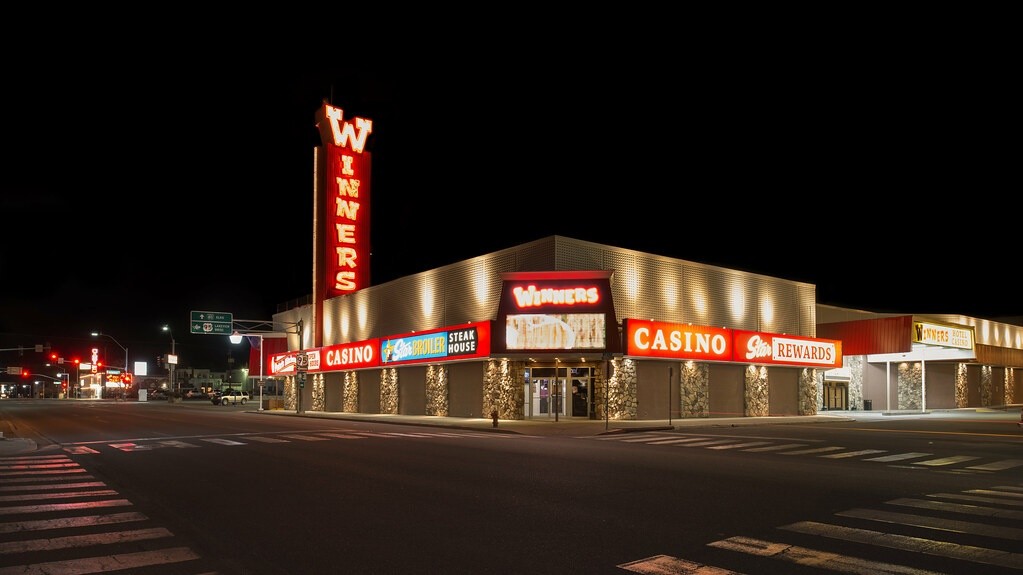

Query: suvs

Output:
[210, 390, 250, 406]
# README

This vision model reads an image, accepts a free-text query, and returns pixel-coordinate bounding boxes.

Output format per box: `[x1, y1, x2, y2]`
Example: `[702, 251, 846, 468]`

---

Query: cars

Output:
[186, 389, 202, 400]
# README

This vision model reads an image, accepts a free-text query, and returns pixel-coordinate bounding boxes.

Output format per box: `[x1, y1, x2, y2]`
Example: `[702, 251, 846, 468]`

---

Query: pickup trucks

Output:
[207, 389, 223, 399]
[150, 389, 171, 400]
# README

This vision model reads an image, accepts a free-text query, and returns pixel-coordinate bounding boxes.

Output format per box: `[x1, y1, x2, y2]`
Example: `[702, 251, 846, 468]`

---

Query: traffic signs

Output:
[191, 311, 233, 323]
[190, 321, 233, 335]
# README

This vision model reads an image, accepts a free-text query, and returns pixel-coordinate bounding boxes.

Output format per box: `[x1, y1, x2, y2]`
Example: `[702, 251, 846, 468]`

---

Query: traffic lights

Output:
[97, 362, 102, 367]
[62, 382, 66, 388]
[23, 372, 28, 376]
[74, 359, 79, 364]
[52, 354, 56, 359]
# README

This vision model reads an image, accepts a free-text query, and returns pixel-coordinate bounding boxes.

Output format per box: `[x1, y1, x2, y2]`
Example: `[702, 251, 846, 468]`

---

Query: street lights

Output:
[229, 331, 266, 411]
[162, 325, 175, 391]
[91, 332, 128, 401]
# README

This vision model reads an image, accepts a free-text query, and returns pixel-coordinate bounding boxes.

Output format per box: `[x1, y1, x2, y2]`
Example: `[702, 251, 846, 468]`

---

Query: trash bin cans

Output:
[262, 399, 274, 410]
[864, 400, 872, 410]
